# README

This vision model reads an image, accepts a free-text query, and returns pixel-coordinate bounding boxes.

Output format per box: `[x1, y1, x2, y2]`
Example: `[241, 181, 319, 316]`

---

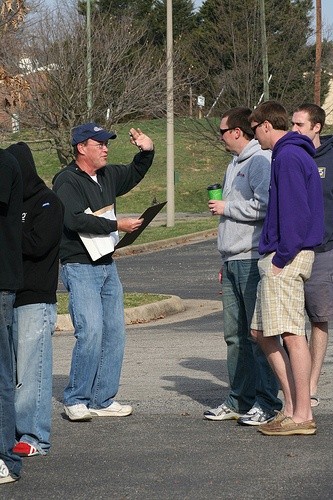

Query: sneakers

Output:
[256, 410, 317, 436]
[89, 400, 133, 416]
[12, 441, 40, 457]
[237, 401, 283, 426]
[204, 402, 246, 420]
[63, 403, 93, 422]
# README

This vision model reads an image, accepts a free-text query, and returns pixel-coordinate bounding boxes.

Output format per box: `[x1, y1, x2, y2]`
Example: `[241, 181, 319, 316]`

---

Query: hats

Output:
[72, 123, 117, 146]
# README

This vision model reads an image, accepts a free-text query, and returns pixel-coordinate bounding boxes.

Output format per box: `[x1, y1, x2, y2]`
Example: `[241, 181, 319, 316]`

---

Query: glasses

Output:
[83, 142, 111, 149]
[220, 128, 234, 135]
[251, 120, 264, 134]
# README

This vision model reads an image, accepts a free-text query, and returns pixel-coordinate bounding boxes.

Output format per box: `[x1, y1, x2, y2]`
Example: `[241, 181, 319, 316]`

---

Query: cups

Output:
[207, 183, 223, 200]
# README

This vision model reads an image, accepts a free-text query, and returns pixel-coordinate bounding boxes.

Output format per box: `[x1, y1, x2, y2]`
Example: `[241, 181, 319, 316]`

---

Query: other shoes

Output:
[0, 459, 17, 484]
[310, 396, 321, 407]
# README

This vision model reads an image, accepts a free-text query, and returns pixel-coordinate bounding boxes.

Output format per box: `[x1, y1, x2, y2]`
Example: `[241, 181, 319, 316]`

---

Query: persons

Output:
[291, 104, 333, 406]
[0, 146, 23, 482]
[202, 108, 283, 427]
[50, 123, 154, 421]
[248, 99, 324, 435]
[6, 141, 66, 458]
